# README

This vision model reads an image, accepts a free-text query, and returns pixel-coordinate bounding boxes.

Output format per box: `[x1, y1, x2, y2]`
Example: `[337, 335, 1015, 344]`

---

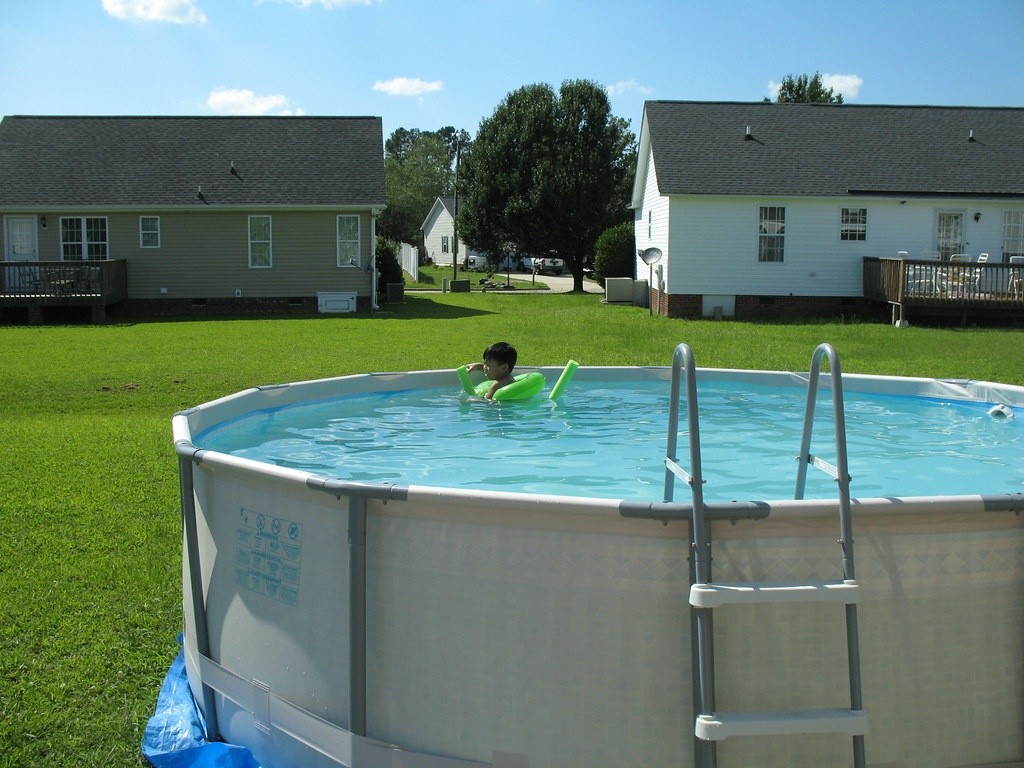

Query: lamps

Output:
[975, 213, 983, 223]
[40, 217, 49, 230]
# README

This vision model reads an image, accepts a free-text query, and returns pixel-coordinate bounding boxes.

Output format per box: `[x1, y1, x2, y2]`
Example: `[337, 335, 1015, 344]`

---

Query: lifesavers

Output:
[456, 359, 579, 401]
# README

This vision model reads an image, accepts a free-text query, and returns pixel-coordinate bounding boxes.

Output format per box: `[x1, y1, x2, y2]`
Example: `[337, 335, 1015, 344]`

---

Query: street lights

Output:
[450, 132, 464, 280]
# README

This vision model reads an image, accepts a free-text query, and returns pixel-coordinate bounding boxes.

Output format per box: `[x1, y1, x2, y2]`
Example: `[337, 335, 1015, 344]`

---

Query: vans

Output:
[519, 247, 565, 277]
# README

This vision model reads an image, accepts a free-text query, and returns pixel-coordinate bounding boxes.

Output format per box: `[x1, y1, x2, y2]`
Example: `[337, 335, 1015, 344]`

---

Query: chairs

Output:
[898, 248, 1024, 301]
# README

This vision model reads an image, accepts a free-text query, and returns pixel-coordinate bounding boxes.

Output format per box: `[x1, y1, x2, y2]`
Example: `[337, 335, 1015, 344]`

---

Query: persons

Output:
[466, 342, 517, 400]
